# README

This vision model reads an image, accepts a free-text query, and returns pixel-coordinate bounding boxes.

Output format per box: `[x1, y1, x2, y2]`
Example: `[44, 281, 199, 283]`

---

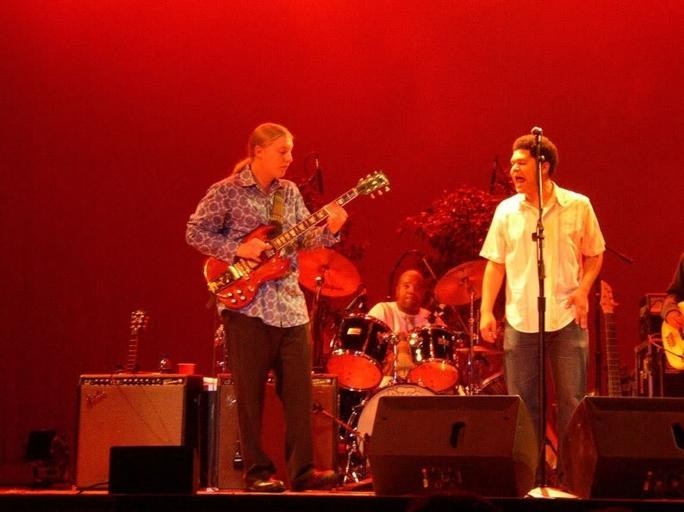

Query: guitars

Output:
[203, 171, 390, 309]
[662, 301, 684, 371]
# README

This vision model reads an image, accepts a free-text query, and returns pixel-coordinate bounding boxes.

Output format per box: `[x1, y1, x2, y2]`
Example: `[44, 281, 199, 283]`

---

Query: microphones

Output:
[344, 288, 368, 312]
[315, 157, 324, 193]
[531, 126, 544, 135]
[488, 154, 499, 193]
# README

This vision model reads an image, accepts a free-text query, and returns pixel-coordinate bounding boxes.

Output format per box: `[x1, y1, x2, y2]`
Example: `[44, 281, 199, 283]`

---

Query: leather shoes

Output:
[247, 476, 287, 492]
[292, 469, 336, 491]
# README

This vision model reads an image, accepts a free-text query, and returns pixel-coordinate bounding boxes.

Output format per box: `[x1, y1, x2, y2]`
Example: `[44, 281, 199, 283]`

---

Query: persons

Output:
[184, 121, 349, 494]
[363, 269, 456, 387]
[478, 131, 608, 501]
[655, 243, 684, 398]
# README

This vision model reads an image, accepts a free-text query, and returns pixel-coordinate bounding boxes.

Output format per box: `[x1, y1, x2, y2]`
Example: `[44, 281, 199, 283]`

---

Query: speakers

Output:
[557, 396, 683, 501]
[368, 397, 537, 498]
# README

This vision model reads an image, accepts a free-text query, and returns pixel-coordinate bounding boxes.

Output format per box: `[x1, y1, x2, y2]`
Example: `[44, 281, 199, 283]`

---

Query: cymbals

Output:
[294, 248, 362, 298]
[433, 259, 487, 306]
[455, 345, 504, 357]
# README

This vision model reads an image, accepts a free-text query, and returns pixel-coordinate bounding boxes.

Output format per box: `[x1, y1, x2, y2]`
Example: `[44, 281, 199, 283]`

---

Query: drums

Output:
[346, 382, 441, 487]
[326, 313, 396, 391]
[391, 324, 464, 393]
[475, 371, 508, 395]
